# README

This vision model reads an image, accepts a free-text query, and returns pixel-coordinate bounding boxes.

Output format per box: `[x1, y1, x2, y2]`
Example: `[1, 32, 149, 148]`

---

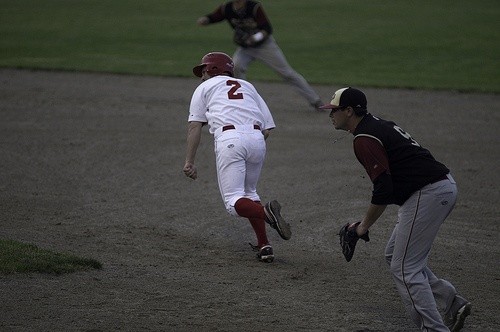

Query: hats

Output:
[319, 87, 367, 109]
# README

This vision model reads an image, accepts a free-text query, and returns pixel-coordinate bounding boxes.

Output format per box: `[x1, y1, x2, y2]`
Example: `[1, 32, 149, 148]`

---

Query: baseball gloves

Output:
[233, 27, 258, 49]
[336, 220, 371, 263]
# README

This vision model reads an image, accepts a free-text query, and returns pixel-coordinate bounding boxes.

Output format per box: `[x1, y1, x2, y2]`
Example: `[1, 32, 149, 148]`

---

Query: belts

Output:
[435, 175, 448, 182]
[223, 125, 261, 132]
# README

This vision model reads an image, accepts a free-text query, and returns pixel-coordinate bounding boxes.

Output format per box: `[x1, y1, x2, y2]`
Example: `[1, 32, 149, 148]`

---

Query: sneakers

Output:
[264, 199, 292, 240]
[248, 242, 274, 263]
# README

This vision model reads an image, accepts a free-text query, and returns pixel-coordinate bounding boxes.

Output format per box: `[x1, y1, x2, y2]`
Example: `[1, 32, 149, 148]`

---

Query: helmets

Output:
[193, 52, 235, 77]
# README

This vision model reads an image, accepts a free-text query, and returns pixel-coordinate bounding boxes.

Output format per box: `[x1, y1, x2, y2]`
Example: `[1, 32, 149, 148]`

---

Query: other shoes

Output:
[314, 99, 324, 111]
[445, 295, 472, 332]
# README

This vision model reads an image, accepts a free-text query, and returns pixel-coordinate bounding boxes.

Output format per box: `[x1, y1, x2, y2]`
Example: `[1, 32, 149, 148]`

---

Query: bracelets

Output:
[185, 160, 194, 164]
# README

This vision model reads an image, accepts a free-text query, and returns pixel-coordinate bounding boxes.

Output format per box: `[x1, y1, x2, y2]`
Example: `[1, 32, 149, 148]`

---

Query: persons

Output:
[184, 52, 292, 261]
[320, 86, 471, 332]
[199, 0, 327, 112]
